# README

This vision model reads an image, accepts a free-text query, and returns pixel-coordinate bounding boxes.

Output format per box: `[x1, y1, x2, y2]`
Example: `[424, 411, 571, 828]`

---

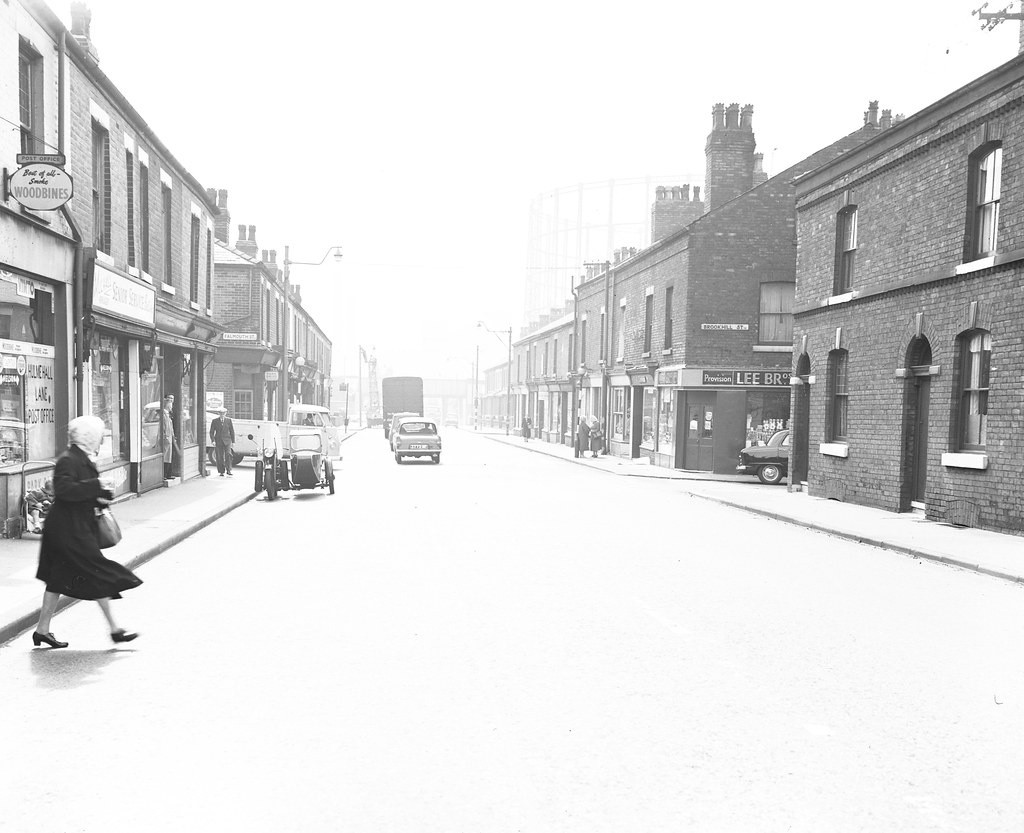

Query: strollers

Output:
[17, 461, 57, 539]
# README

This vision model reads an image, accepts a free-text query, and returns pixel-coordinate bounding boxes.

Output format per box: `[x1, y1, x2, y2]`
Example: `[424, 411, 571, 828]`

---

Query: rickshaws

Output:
[255, 430, 336, 502]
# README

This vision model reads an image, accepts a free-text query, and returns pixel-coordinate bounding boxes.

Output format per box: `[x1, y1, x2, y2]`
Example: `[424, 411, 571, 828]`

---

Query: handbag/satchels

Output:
[97, 508, 122, 548]
[592, 430, 604, 440]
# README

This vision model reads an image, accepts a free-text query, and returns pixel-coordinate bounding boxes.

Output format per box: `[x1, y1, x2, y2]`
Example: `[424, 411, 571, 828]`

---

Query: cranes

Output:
[367, 356, 381, 407]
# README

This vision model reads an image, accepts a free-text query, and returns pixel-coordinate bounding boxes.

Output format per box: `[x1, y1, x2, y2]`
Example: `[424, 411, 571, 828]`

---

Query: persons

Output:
[419, 423, 433, 432]
[209, 408, 236, 476]
[521, 414, 532, 442]
[301, 413, 316, 426]
[578, 415, 602, 458]
[163, 393, 177, 480]
[33, 416, 143, 648]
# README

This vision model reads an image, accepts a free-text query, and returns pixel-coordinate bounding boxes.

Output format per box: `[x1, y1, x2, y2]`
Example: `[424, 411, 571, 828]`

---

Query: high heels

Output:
[111, 631, 138, 642]
[33, 632, 68, 647]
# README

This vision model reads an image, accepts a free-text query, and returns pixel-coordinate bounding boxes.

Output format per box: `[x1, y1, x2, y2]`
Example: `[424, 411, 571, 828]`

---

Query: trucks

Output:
[202, 403, 343, 467]
[365, 405, 384, 428]
[382, 377, 424, 425]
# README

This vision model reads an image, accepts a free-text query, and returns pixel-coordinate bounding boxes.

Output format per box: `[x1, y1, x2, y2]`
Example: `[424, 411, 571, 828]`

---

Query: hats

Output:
[219, 408, 227, 412]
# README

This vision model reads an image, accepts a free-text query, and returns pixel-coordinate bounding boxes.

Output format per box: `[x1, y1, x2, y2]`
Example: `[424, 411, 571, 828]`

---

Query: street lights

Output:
[295, 356, 306, 403]
[474, 319, 512, 436]
[280, 243, 344, 422]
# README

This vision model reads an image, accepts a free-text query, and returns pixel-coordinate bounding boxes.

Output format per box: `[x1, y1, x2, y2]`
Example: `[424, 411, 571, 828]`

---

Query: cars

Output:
[445, 413, 458, 428]
[736, 428, 790, 486]
[383, 412, 442, 465]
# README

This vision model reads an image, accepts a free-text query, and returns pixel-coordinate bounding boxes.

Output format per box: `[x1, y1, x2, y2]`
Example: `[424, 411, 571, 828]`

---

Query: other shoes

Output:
[227, 473, 232, 475]
[167, 477, 175, 479]
[592, 455, 598, 458]
[220, 474, 224, 476]
